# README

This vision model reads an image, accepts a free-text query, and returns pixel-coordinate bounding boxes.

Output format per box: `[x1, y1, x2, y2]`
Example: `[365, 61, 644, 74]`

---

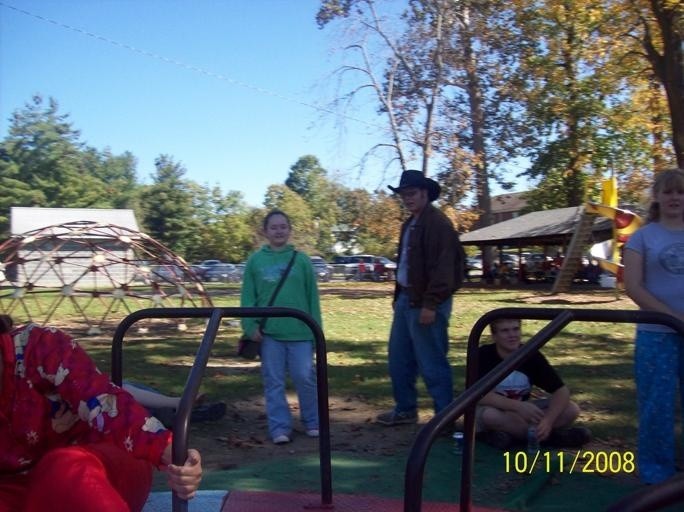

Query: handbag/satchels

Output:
[237, 335, 259, 361]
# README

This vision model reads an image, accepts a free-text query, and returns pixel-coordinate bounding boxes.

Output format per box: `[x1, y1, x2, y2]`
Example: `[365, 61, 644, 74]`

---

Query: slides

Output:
[584, 178, 645, 292]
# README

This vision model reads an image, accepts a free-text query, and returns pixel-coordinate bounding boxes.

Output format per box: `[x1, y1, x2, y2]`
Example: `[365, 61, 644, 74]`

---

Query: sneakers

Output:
[554, 425, 592, 447]
[306, 430, 319, 438]
[485, 428, 520, 450]
[375, 409, 419, 426]
[273, 435, 290, 443]
[189, 400, 227, 423]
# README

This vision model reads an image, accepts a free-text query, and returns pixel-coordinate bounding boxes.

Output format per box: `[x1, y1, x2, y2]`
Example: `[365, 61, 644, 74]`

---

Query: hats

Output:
[386, 170, 441, 202]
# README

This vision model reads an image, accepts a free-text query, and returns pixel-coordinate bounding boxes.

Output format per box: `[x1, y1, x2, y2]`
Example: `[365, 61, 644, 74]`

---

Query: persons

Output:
[375, 170, 466, 437]
[121, 379, 226, 428]
[240, 210, 324, 444]
[122, 383, 205, 409]
[476, 316, 592, 453]
[622, 169, 683, 486]
[0, 313, 201, 511]
[357, 259, 365, 280]
[373, 257, 384, 280]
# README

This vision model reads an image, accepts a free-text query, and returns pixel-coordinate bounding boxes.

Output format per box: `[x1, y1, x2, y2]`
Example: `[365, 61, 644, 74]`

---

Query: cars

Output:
[309, 255, 398, 281]
[149, 259, 247, 282]
[468, 254, 590, 283]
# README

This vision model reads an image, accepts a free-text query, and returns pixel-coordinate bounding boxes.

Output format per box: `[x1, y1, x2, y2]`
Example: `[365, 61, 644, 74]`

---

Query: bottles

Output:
[527, 419, 540, 454]
